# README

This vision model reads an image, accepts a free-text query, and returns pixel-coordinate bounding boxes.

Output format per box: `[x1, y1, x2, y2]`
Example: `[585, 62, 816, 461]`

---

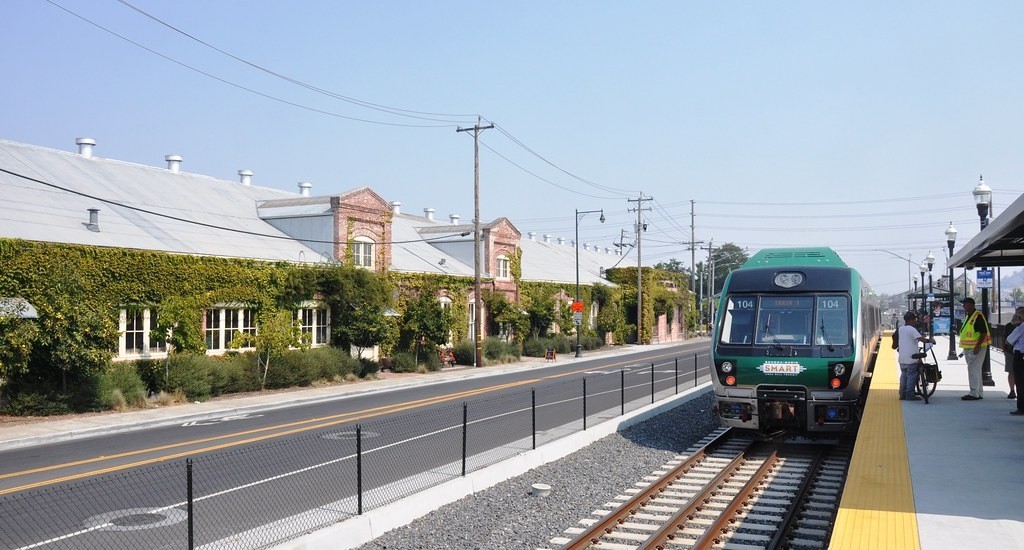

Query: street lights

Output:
[913, 272, 918, 314]
[919, 261, 928, 337]
[571, 208, 606, 359]
[944, 221, 958, 360]
[924, 249, 937, 345]
[971, 174, 995, 385]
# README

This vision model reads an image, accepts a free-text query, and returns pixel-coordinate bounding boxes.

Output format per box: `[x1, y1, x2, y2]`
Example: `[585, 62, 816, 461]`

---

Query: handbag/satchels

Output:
[925, 364, 940, 383]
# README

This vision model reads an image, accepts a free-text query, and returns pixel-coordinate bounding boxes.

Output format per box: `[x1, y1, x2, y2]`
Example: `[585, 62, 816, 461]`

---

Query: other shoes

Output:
[899, 396, 922, 402]
[1008, 392, 1016, 398]
[1011, 409, 1024, 416]
[961, 395, 983, 400]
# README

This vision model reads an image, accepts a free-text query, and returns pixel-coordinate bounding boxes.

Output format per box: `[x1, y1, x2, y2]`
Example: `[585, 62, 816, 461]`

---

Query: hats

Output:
[904, 311, 917, 320]
[958, 298, 975, 305]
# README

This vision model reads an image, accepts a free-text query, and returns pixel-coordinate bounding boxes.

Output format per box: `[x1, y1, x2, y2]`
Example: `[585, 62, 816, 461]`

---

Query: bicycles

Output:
[911, 346, 937, 404]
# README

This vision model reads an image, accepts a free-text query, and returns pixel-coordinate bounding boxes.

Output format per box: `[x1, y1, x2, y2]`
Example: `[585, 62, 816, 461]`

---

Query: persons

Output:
[1003, 307, 1024, 414]
[959, 298, 992, 400]
[1002, 306, 1022, 399]
[897, 311, 936, 401]
[912, 309, 931, 335]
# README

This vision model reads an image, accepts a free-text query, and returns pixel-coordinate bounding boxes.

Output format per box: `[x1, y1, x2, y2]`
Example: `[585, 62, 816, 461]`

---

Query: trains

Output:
[708, 245, 883, 450]
